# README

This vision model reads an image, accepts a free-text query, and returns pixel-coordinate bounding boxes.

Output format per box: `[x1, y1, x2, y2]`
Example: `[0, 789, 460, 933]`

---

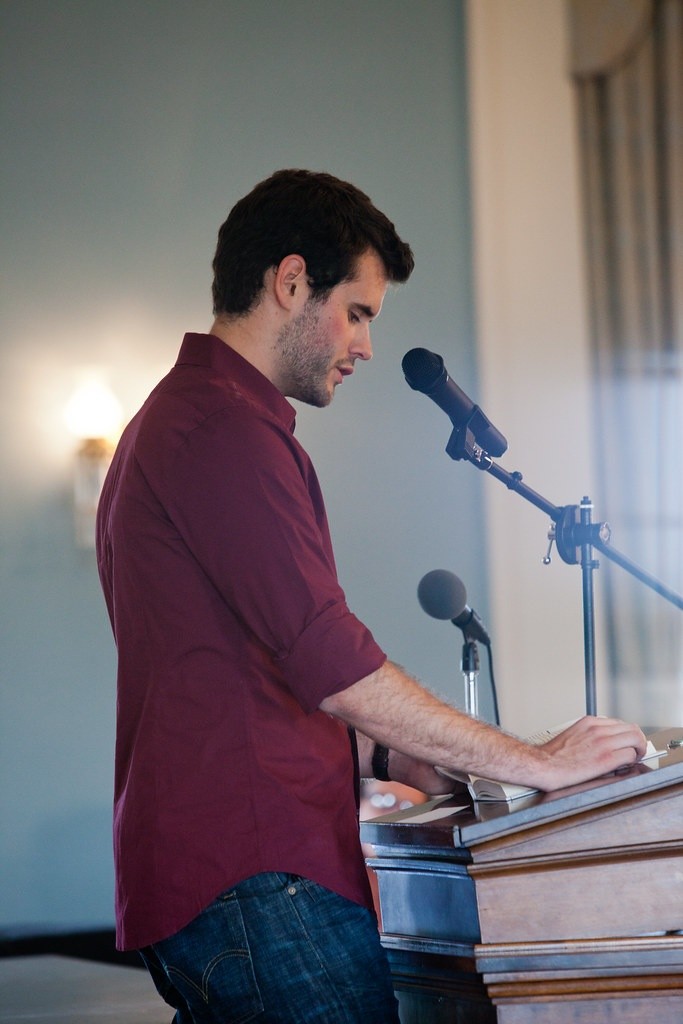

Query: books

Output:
[435, 716, 667, 802]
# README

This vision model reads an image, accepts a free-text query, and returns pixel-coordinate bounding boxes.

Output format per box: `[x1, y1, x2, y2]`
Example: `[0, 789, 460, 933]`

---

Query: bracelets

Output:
[371, 740, 390, 782]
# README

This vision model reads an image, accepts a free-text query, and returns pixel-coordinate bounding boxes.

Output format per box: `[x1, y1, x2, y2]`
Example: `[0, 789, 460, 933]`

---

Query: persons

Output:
[94, 168, 648, 1024]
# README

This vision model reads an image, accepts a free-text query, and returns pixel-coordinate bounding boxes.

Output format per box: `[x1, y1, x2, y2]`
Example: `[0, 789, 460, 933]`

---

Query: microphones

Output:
[418, 568, 491, 646]
[402, 348, 508, 458]
